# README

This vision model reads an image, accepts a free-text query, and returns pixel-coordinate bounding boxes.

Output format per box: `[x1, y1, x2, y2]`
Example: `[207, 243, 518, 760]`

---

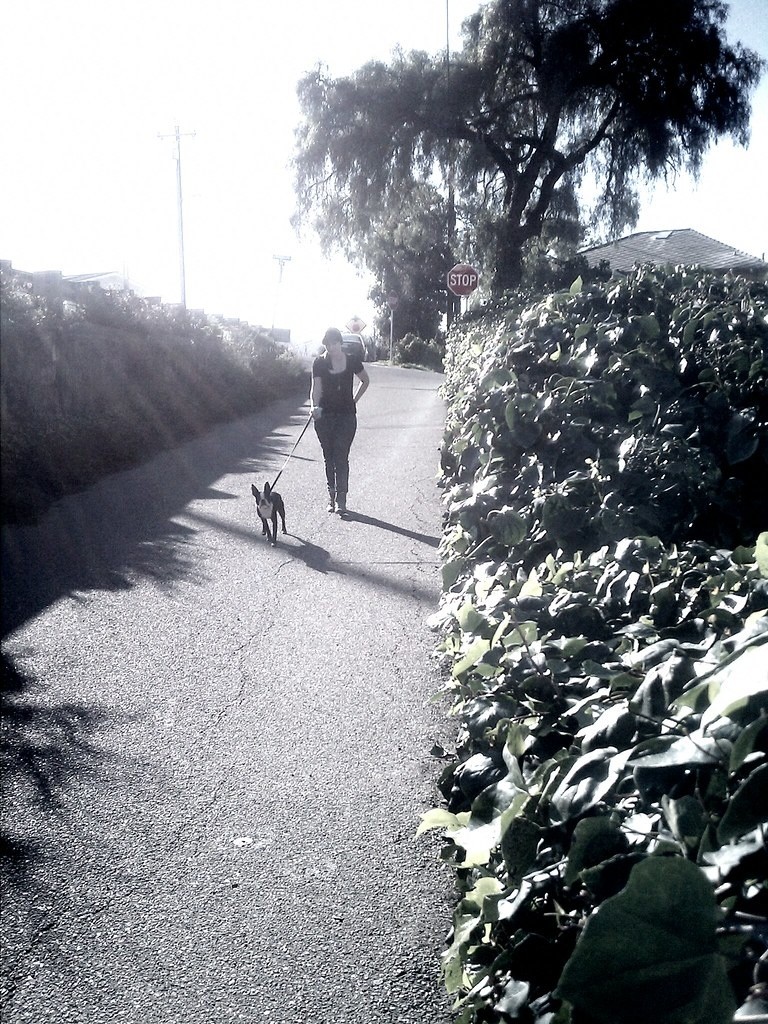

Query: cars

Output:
[339, 332, 368, 363]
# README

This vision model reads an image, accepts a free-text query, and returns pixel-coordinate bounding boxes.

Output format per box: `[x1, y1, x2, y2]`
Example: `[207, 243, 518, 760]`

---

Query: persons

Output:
[309, 327, 370, 514]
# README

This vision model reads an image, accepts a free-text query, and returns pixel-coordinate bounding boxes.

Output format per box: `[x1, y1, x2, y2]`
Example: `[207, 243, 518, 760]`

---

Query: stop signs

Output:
[446, 263, 479, 297]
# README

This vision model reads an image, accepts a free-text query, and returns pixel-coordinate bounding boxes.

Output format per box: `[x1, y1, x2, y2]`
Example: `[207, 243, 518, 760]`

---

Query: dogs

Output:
[251, 482, 288, 546]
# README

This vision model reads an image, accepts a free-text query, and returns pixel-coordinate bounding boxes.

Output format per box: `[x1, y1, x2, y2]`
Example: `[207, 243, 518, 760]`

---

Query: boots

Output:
[326, 488, 347, 516]
